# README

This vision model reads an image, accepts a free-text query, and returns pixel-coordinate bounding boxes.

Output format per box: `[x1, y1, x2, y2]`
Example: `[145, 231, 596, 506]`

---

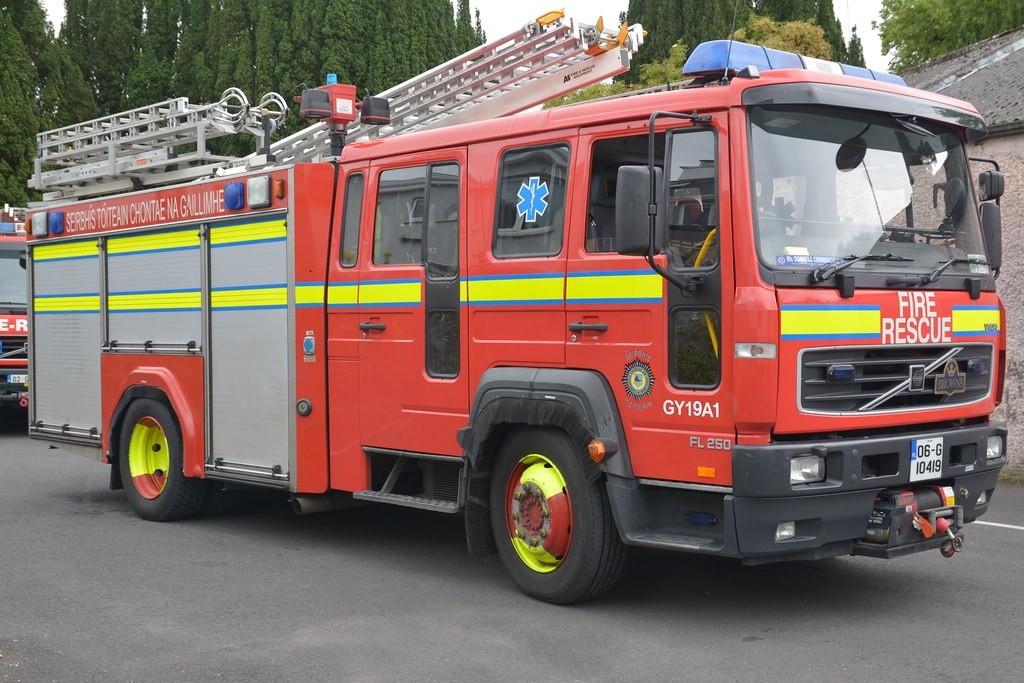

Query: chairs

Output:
[587, 169, 617, 253]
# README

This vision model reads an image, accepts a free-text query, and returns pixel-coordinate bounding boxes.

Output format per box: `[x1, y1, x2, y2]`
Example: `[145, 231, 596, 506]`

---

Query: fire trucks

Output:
[0, 9, 1009, 605]
